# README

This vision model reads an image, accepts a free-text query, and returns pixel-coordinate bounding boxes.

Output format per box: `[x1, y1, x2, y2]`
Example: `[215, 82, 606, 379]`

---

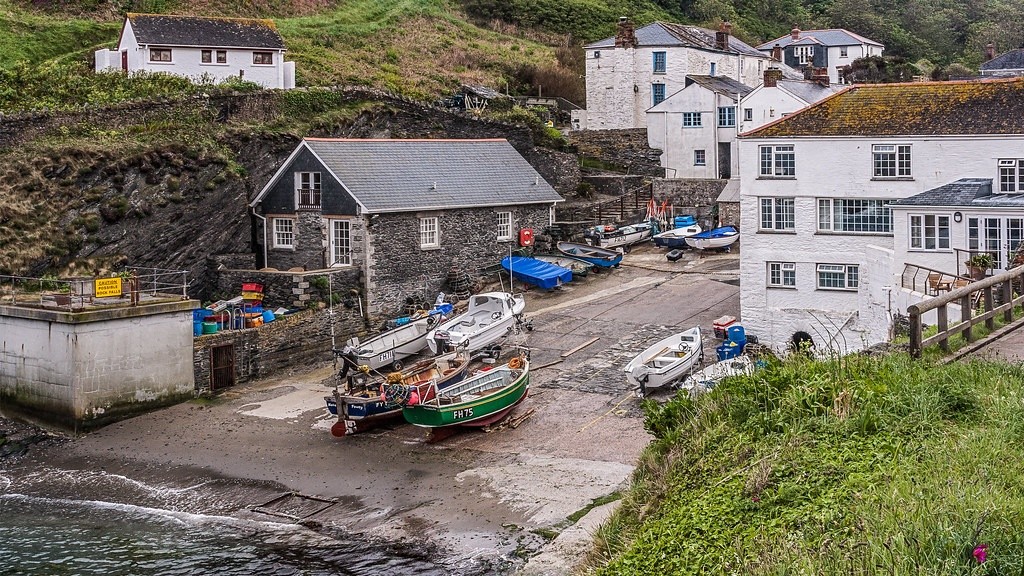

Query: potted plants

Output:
[964, 254, 996, 281]
[115, 270, 135, 292]
[53, 283, 70, 306]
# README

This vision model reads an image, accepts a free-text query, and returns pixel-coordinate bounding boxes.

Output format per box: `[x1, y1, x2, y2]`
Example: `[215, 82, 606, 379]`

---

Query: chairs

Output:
[928, 273, 969, 305]
[260, 266, 278, 272]
[288, 267, 304, 272]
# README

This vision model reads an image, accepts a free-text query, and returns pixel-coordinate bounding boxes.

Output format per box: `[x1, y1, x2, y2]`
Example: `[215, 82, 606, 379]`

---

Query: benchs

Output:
[655, 357, 680, 362]
[448, 331, 463, 341]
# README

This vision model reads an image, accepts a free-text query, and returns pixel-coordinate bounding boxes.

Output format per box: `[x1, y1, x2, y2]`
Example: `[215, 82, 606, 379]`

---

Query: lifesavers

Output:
[604, 226, 615, 232]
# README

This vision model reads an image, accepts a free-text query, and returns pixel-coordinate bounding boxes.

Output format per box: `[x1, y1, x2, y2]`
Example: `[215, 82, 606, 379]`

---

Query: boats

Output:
[622, 325, 704, 399]
[557, 240, 622, 267]
[535, 256, 595, 277]
[404, 344, 529, 427]
[330, 344, 468, 422]
[680, 354, 753, 403]
[333, 300, 454, 374]
[426, 245, 526, 357]
[684, 225, 740, 250]
[652, 223, 702, 248]
[585, 222, 654, 248]
[501, 255, 573, 290]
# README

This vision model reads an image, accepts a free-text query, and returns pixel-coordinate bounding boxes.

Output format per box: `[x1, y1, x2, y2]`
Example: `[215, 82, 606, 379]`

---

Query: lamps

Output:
[954, 212, 962, 223]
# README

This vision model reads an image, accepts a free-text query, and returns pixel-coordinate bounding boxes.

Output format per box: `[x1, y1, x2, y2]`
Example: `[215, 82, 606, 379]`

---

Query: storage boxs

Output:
[194, 282, 265, 328]
[585, 251, 597, 255]
